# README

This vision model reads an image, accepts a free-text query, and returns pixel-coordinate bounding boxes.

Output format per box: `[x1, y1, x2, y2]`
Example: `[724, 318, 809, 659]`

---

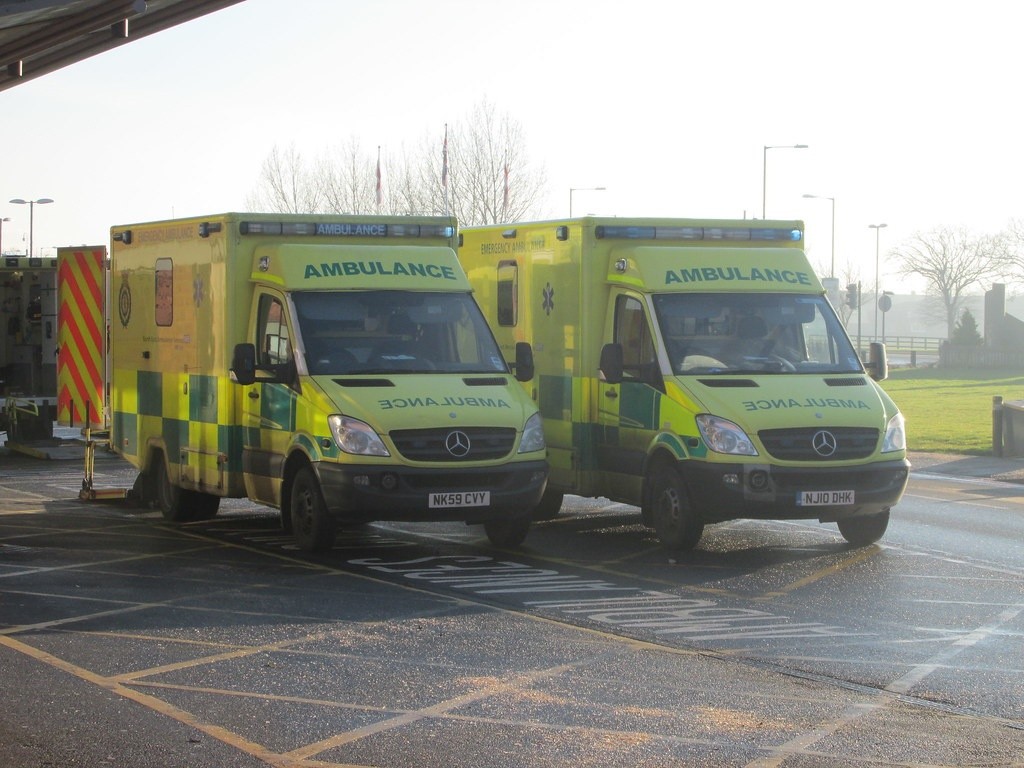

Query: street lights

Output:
[867, 223, 889, 343]
[569, 186, 607, 219]
[762, 143, 809, 221]
[8, 198, 55, 259]
[0, 217, 12, 258]
[881, 290, 895, 346]
[802, 194, 835, 277]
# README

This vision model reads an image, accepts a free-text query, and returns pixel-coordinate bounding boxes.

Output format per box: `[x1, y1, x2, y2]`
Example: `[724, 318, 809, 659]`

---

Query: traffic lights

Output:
[845, 284, 857, 310]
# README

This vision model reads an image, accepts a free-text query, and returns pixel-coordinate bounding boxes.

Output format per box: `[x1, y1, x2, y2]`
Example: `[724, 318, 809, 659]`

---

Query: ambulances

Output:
[51, 210, 552, 557]
[455, 215, 913, 558]
